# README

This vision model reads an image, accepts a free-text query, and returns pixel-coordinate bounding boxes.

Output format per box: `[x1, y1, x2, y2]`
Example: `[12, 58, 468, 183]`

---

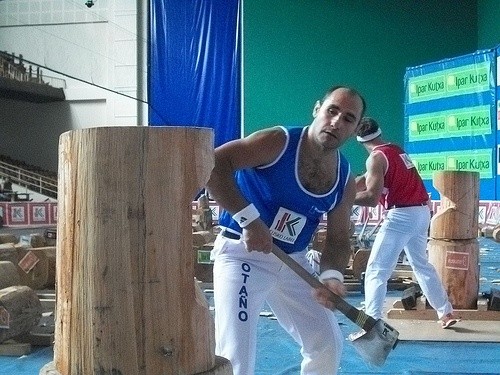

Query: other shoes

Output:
[442, 316, 457, 329]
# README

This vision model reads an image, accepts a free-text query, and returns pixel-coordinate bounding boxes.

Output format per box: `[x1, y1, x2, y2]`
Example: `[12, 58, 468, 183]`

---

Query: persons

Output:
[350, 116, 458, 342]
[204, 86, 366, 375]
[0, 175, 13, 201]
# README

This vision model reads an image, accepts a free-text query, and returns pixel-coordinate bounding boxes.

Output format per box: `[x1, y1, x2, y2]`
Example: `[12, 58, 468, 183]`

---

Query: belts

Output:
[390, 202, 428, 209]
[220, 229, 240, 240]
